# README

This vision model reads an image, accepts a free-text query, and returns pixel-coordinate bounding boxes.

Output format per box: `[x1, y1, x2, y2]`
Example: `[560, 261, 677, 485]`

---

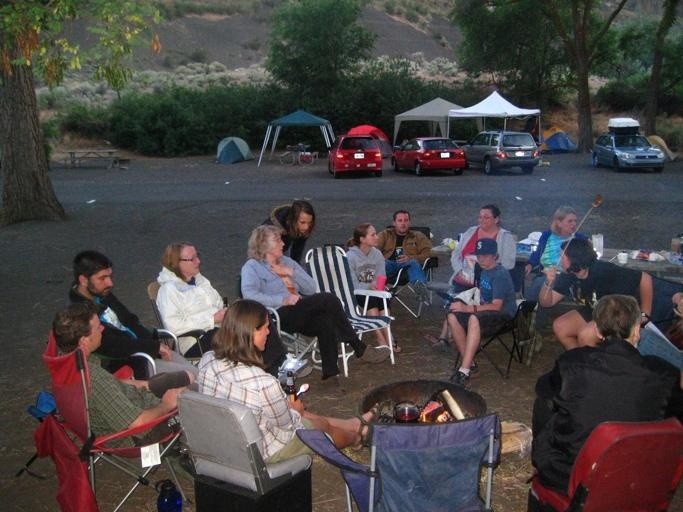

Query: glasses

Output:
[179, 254, 199, 262]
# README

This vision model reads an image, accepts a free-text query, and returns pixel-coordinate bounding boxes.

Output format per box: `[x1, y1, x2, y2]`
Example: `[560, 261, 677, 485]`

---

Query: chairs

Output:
[234, 275, 314, 378]
[177, 384, 312, 511]
[305, 244, 396, 378]
[527, 418, 683, 512]
[42, 330, 184, 512]
[507, 257, 528, 300]
[382, 226, 438, 317]
[149, 282, 204, 361]
[448, 300, 537, 379]
[297, 411, 502, 512]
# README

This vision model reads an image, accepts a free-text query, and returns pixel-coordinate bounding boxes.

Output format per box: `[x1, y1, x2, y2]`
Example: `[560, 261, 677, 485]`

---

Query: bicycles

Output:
[280, 143, 313, 167]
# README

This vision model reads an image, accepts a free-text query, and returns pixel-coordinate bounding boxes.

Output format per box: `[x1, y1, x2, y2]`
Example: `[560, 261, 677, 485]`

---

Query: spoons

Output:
[297, 382, 310, 397]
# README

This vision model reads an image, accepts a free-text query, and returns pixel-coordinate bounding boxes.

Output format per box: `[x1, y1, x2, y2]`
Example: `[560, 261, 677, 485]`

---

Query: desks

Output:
[432, 244, 683, 310]
[63, 150, 118, 169]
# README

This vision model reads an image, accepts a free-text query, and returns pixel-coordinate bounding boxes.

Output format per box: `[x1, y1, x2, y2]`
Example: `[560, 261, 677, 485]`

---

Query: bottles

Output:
[223, 296, 227, 309]
[154, 478, 183, 511]
[285, 371, 297, 405]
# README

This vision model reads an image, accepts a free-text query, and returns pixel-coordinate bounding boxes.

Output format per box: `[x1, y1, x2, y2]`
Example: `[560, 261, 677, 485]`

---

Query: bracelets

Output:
[544, 281, 552, 290]
[474, 305, 478, 313]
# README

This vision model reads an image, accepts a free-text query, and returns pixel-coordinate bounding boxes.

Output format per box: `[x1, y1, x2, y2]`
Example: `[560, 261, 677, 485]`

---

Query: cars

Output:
[327, 136, 383, 177]
[592, 118, 665, 171]
[391, 136, 465, 176]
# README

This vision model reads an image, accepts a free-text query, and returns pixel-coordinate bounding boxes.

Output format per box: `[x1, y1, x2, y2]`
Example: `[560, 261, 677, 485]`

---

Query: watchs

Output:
[642, 312, 650, 319]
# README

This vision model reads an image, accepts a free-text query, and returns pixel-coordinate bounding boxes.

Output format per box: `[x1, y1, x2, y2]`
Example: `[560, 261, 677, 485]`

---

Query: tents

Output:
[538, 125, 578, 153]
[648, 135, 675, 162]
[215, 136, 255, 166]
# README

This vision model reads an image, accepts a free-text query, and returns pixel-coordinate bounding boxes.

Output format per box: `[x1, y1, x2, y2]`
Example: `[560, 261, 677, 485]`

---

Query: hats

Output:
[469, 238, 498, 255]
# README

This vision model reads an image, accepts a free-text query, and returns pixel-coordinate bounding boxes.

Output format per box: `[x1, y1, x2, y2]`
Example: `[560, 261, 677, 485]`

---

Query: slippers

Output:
[392, 343, 401, 354]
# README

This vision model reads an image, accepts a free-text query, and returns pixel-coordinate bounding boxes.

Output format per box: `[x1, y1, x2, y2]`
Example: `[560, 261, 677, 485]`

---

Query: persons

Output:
[156, 241, 288, 380]
[438, 204, 516, 350]
[522, 204, 586, 355]
[530, 295, 683, 493]
[345, 223, 402, 355]
[239, 222, 391, 383]
[51, 301, 198, 449]
[260, 199, 317, 264]
[446, 237, 517, 387]
[197, 298, 380, 465]
[536, 237, 654, 353]
[69, 249, 200, 383]
[375, 209, 435, 303]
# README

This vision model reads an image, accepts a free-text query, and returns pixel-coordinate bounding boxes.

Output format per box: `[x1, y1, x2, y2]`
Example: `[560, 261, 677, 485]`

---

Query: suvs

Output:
[462, 130, 539, 173]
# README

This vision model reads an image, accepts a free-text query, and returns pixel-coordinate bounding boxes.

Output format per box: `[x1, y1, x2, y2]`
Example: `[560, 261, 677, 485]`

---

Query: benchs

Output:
[275, 145, 319, 167]
[74, 157, 115, 169]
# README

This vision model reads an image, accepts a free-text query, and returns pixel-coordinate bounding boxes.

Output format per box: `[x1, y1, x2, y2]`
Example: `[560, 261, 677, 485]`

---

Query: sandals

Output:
[356, 401, 384, 445]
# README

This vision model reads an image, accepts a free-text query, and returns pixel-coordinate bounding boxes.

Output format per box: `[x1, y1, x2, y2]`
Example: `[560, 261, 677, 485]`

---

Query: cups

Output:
[376, 275, 387, 290]
[618, 253, 628, 265]
[457, 233, 464, 242]
[395, 247, 404, 259]
[592, 234, 603, 257]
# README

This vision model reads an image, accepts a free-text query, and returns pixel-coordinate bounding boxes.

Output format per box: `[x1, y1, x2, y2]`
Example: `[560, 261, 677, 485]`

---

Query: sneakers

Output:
[358, 344, 391, 364]
[277, 352, 347, 398]
[448, 362, 480, 392]
[415, 280, 427, 301]
[424, 333, 451, 353]
